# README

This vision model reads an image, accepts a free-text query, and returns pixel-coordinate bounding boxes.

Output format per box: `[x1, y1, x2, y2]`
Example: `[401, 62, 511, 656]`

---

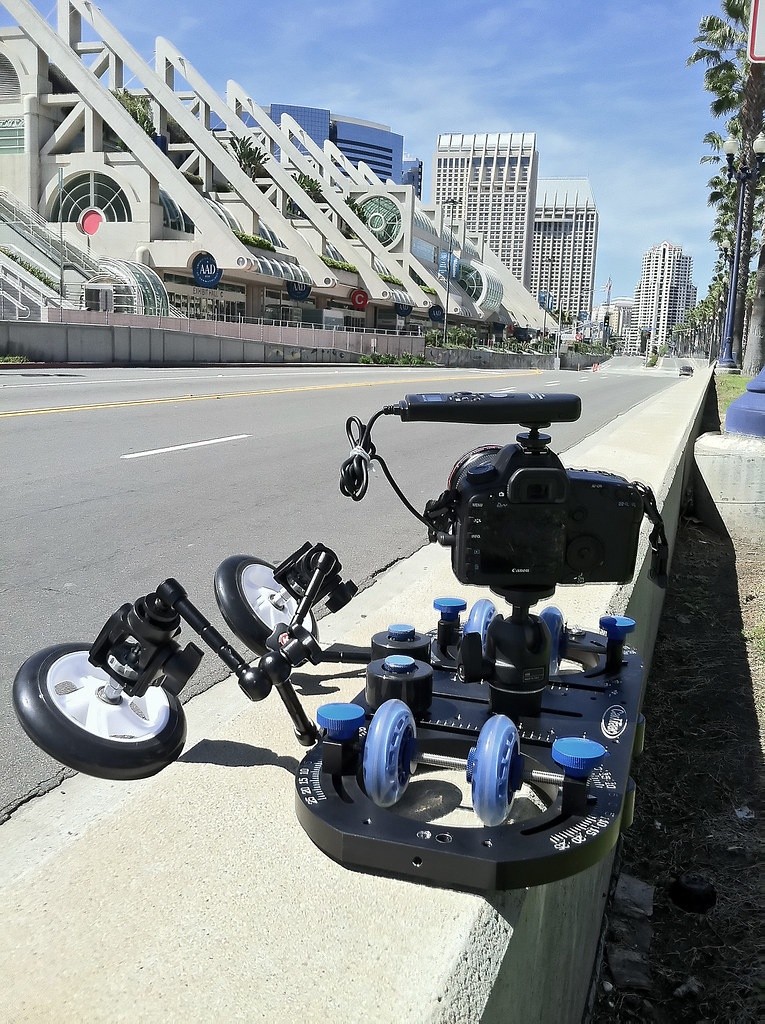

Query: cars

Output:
[679, 366, 695, 376]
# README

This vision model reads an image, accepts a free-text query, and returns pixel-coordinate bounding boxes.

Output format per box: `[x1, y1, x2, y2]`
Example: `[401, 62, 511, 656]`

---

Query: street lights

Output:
[718, 236, 735, 364]
[717, 132, 765, 368]
[442, 198, 459, 343]
[541, 257, 556, 355]
